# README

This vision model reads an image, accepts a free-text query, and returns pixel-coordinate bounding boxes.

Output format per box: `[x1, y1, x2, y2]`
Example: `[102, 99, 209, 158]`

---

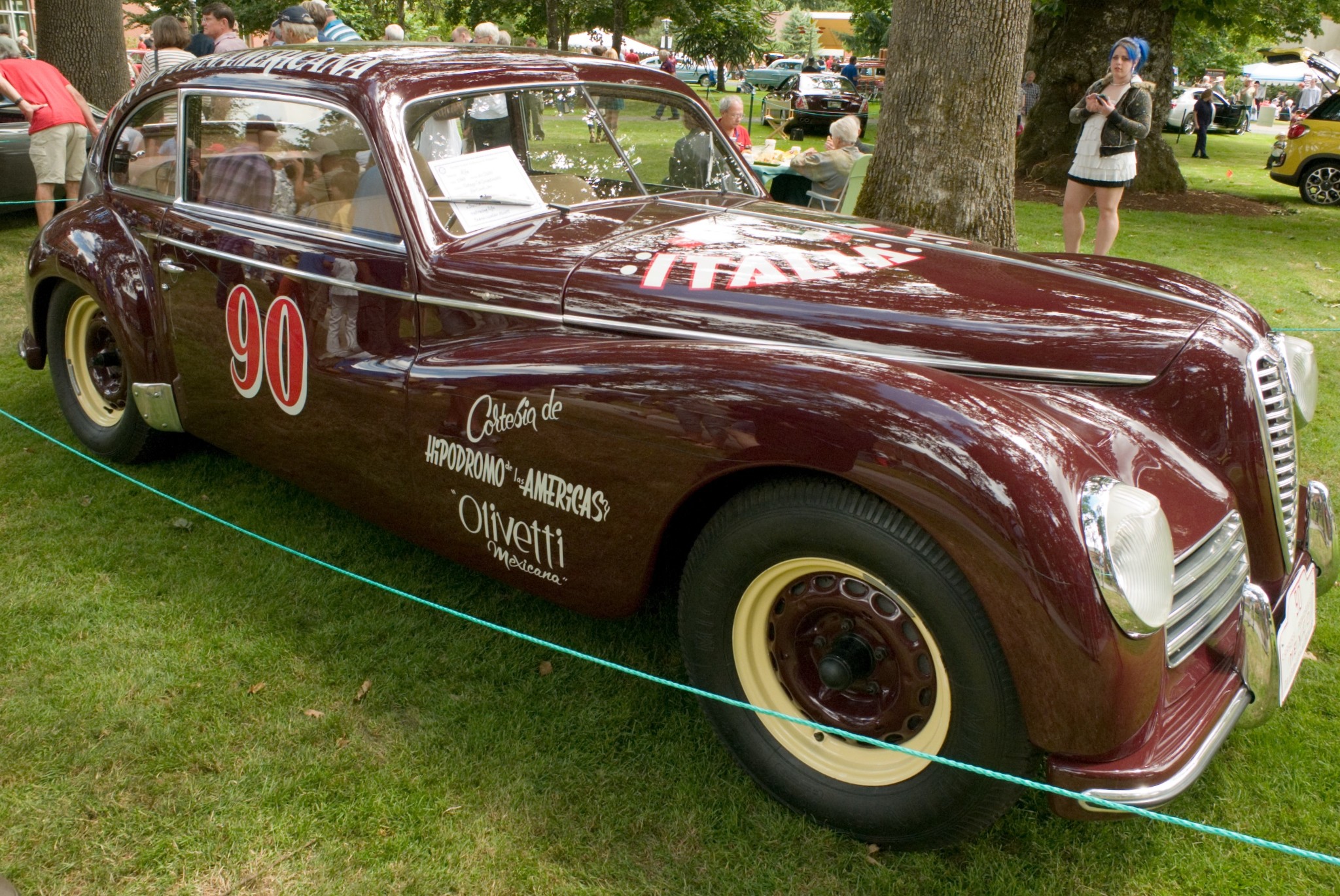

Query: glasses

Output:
[201, 17, 218, 24]
[474, 35, 490, 41]
[727, 113, 746, 120]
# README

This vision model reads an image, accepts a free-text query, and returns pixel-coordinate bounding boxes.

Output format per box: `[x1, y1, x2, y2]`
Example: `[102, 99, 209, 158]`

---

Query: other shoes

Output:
[534, 135, 545, 141]
[590, 139, 595, 143]
[1265, 165, 1272, 169]
[1245, 129, 1249, 132]
[668, 116, 680, 120]
[596, 137, 601, 142]
[1192, 154, 1198, 158]
[651, 114, 661, 120]
[1200, 154, 1208, 158]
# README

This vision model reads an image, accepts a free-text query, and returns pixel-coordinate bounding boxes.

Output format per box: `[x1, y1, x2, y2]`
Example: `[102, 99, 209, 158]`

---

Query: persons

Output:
[132, 16, 199, 90]
[124, 0, 366, 90]
[200, 2, 250, 56]
[378, 0, 1340, 267]
[1192, 88, 1213, 159]
[1063, 36, 1152, 258]
[117, 112, 409, 361]
[769, 113, 861, 214]
[0, 23, 100, 233]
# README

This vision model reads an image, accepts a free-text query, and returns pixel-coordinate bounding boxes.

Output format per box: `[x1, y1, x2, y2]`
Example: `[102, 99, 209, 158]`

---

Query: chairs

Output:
[806, 154, 873, 213]
[764, 100, 794, 141]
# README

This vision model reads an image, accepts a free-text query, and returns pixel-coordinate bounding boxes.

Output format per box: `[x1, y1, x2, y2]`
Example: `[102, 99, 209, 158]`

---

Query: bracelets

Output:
[13, 98, 26, 108]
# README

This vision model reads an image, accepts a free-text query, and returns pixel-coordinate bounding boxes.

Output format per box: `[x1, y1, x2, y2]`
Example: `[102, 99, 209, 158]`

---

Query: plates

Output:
[753, 160, 781, 166]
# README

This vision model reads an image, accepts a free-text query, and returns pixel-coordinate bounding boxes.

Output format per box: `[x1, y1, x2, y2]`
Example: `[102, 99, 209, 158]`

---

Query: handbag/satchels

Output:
[432, 99, 464, 121]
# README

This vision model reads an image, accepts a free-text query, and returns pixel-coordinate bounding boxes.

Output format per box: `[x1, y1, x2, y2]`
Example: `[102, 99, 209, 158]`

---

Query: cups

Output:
[791, 146, 801, 156]
[765, 139, 777, 161]
[751, 145, 766, 161]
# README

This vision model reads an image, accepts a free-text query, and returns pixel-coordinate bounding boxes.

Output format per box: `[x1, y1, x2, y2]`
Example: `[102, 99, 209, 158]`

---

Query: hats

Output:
[139, 34, 145, 39]
[808, 56, 817, 63]
[1275, 133, 1287, 139]
[312, 135, 342, 156]
[271, 7, 314, 28]
[244, 115, 276, 133]
[312, 0, 332, 10]
[1213, 76, 1226, 83]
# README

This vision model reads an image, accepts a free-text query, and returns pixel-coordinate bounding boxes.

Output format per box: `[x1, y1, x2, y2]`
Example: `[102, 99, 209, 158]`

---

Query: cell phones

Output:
[1097, 95, 1108, 106]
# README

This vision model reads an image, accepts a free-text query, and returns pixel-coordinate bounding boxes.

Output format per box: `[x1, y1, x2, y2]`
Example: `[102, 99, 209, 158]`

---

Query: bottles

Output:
[729, 137, 740, 151]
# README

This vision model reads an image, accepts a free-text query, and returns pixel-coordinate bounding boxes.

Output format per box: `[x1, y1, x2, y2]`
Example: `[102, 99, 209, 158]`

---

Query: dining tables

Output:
[740, 148, 802, 187]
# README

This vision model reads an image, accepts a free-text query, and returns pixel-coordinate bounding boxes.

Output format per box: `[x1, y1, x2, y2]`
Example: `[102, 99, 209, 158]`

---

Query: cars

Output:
[1165, 87, 1250, 136]
[128, 49, 147, 73]
[0, 103, 109, 217]
[744, 58, 805, 92]
[854, 62, 886, 90]
[21, 41, 1339, 854]
[1257, 46, 1340, 205]
[638, 55, 728, 87]
[760, 73, 869, 138]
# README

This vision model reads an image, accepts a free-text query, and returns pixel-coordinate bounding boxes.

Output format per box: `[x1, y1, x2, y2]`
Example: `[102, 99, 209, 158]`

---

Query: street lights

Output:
[661, 18, 673, 51]
